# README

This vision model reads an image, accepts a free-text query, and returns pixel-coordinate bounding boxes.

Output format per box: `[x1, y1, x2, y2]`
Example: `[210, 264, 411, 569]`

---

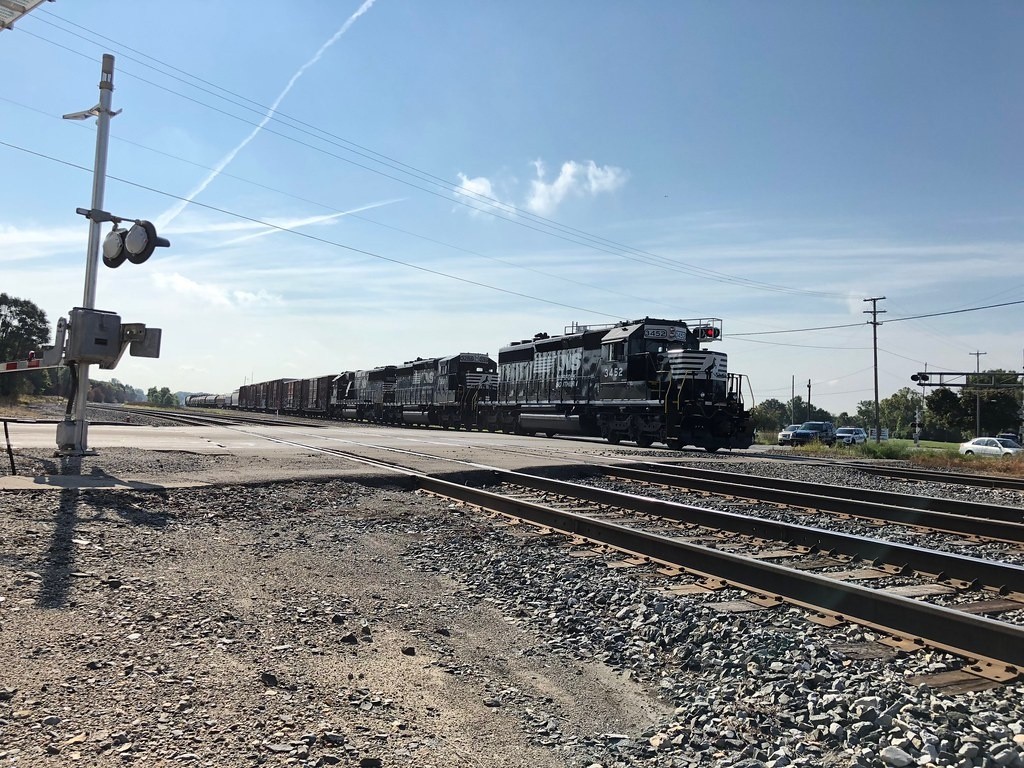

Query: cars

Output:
[959, 437, 1024, 459]
[778, 425, 801, 446]
[836, 428, 867, 446]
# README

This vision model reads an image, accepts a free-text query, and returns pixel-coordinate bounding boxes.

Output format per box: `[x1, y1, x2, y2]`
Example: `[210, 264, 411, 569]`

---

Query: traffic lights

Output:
[918, 423, 924, 428]
[911, 423, 916, 427]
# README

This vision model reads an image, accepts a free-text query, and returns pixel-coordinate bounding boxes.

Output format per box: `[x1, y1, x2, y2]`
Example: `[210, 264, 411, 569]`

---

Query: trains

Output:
[185, 316, 758, 452]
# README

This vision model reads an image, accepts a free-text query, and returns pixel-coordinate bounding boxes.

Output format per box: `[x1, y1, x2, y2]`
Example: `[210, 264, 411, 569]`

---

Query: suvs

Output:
[790, 420, 836, 448]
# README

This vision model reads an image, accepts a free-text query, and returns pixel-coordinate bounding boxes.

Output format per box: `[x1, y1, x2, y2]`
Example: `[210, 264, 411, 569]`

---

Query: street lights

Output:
[807, 379, 811, 422]
[53, 54, 122, 457]
